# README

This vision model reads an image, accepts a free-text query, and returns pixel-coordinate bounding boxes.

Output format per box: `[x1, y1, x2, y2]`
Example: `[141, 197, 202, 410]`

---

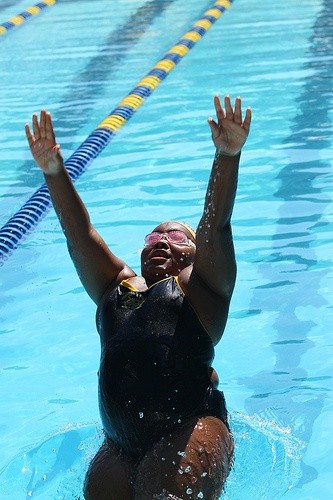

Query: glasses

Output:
[143, 230, 192, 247]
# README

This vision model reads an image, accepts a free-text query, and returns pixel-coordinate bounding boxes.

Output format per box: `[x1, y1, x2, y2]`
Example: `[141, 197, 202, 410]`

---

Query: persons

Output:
[24, 95, 252, 499]
